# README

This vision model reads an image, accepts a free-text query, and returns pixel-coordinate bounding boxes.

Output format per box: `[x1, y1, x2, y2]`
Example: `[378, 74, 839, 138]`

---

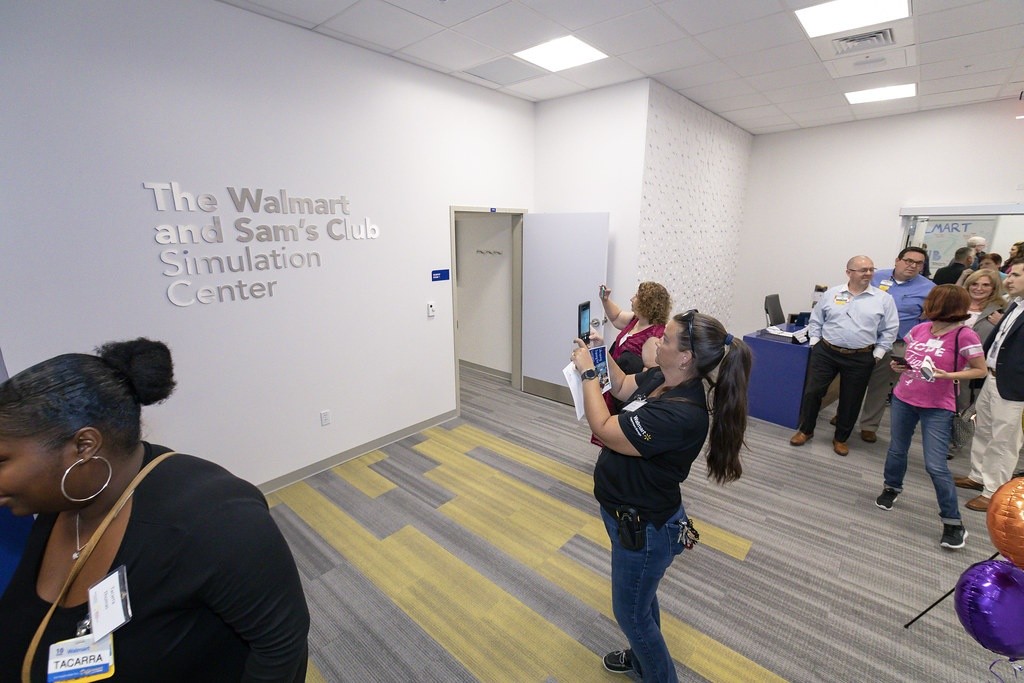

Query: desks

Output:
[743, 322, 840, 430]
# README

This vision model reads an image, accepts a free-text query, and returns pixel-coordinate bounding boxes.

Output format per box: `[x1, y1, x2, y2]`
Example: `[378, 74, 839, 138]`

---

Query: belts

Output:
[877, 335, 904, 343]
[823, 338, 874, 354]
[989, 367, 996, 377]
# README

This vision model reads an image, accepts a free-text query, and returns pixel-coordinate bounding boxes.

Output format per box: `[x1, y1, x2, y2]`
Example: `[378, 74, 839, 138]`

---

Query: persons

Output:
[575, 309, 752, 683]
[831, 247, 939, 442]
[0, 336, 312, 683]
[922, 237, 1024, 511]
[591, 282, 674, 447]
[790, 255, 900, 455]
[875, 284, 987, 549]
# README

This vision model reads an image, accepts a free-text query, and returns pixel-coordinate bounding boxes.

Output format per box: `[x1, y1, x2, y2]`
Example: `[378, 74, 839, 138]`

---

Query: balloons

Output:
[987, 477, 1024, 567]
[954, 561, 1024, 672]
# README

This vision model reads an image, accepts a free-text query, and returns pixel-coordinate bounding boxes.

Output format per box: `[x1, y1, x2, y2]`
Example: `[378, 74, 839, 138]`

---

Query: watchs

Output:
[581, 369, 600, 382]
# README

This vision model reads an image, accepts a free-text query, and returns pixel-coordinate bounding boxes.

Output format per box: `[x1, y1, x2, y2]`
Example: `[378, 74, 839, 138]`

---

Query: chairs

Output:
[765, 293, 785, 328]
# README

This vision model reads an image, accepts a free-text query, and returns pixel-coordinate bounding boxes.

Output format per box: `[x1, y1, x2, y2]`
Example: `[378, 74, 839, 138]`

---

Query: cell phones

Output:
[601, 287, 605, 299]
[890, 355, 913, 370]
[579, 301, 590, 345]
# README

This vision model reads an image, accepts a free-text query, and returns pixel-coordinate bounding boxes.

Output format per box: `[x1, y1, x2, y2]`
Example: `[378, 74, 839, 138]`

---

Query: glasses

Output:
[849, 268, 876, 272]
[900, 258, 924, 266]
[682, 308, 699, 359]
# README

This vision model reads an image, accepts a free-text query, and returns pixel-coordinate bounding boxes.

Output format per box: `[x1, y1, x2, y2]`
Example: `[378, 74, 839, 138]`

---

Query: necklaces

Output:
[72, 492, 131, 560]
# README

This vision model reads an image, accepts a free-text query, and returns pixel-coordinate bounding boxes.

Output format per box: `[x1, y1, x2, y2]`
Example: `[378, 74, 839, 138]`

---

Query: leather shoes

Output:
[953, 476, 984, 491]
[833, 438, 848, 456]
[965, 494, 991, 511]
[790, 431, 814, 445]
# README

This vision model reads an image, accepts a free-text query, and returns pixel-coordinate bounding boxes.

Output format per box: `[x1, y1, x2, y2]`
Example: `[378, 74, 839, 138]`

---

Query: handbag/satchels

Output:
[951, 402, 978, 448]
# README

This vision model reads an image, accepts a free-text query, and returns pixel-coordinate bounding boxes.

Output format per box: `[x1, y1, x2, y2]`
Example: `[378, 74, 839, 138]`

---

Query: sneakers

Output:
[602, 649, 637, 674]
[940, 520, 969, 548]
[875, 487, 897, 510]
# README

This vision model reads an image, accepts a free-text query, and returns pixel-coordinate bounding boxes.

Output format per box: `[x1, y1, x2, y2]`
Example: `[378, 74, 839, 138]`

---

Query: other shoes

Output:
[947, 443, 961, 460]
[830, 414, 837, 426]
[861, 429, 877, 443]
